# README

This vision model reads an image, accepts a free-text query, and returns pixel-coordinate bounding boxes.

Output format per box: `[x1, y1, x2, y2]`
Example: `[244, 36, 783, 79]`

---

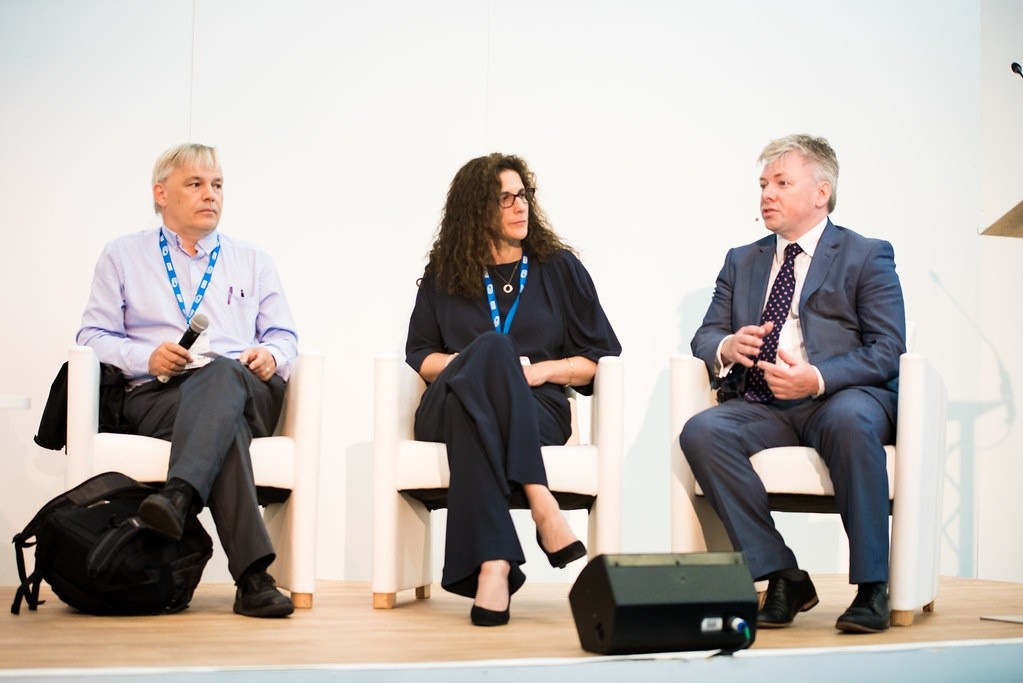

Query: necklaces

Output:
[493, 252, 523, 293]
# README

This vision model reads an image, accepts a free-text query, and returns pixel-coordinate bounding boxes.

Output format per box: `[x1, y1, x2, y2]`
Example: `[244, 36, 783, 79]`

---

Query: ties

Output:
[744, 244, 803, 402]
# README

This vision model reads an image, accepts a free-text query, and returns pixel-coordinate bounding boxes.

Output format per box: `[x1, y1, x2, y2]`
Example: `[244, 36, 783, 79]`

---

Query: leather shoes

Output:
[835, 582, 892, 633]
[138, 482, 191, 540]
[233, 571, 294, 618]
[758, 570, 818, 627]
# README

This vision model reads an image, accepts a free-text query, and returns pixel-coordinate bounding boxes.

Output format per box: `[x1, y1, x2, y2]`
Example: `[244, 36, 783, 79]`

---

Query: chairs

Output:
[370, 350, 624, 610]
[669, 350, 939, 625]
[67, 344, 321, 608]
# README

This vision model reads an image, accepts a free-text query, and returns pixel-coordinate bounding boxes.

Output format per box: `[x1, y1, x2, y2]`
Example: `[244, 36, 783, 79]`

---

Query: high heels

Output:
[471, 565, 511, 625]
[536, 525, 586, 570]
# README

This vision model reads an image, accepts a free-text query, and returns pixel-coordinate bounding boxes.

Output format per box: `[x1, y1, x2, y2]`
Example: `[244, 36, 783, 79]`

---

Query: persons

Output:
[679, 134, 906, 634]
[75, 142, 301, 620]
[406, 152, 623, 627]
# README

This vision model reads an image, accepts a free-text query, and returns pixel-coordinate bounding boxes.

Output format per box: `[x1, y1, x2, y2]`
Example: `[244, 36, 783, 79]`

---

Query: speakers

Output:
[568, 554, 760, 657]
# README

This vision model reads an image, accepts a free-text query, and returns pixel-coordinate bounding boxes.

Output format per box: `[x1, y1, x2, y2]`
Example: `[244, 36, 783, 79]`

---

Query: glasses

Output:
[498, 188, 536, 209]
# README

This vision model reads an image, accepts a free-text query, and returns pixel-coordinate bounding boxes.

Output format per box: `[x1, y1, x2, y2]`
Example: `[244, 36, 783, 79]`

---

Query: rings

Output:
[267, 367, 270, 372]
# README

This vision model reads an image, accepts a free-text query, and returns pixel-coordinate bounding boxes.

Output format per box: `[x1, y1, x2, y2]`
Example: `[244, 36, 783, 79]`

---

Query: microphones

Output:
[155, 313, 209, 384]
[1011, 62, 1023, 78]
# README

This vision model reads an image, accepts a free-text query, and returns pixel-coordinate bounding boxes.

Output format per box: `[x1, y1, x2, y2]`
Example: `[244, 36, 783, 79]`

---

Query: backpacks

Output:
[15, 470, 214, 616]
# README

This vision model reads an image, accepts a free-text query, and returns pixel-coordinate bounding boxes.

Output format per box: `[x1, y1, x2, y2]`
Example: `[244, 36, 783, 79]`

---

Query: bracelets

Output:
[564, 357, 575, 388]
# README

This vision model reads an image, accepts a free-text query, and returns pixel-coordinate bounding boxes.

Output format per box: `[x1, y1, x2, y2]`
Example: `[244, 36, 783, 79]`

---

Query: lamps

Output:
[566, 551, 760, 655]
